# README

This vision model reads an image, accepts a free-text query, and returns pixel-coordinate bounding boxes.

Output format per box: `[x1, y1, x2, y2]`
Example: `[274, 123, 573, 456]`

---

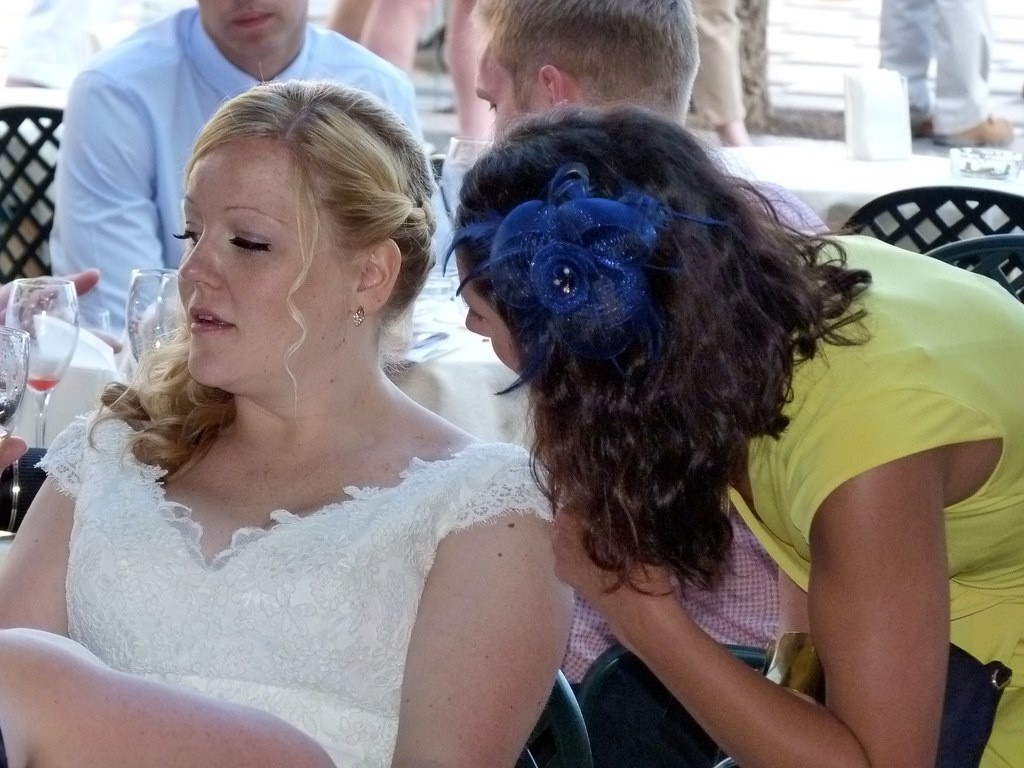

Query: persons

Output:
[0, 0, 1024, 768]
[0, 79, 572, 768]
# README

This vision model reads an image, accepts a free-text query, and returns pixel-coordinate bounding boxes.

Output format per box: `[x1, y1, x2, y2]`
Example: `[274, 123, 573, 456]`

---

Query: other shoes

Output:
[932, 114, 1013, 147]
[910, 117, 932, 138]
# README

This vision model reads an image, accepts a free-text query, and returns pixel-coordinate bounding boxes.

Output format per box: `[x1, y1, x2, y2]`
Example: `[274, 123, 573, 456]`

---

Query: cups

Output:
[127, 267, 182, 364]
[0, 324, 30, 457]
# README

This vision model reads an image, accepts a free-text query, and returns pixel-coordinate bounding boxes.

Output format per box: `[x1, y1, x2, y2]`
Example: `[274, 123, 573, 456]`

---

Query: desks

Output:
[714, 148, 1024, 303]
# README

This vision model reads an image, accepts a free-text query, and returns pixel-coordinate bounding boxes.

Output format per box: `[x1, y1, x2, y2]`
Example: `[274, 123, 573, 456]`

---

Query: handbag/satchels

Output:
[812, 642, 1013, 768]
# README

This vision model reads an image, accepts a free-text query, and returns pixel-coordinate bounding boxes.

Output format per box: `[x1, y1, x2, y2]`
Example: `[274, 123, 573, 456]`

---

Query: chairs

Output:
[839, 185, 1024, 303]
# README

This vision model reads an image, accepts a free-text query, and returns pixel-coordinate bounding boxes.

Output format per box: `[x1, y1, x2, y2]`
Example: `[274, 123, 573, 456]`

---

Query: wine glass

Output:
[4, 277, 80, 448]
